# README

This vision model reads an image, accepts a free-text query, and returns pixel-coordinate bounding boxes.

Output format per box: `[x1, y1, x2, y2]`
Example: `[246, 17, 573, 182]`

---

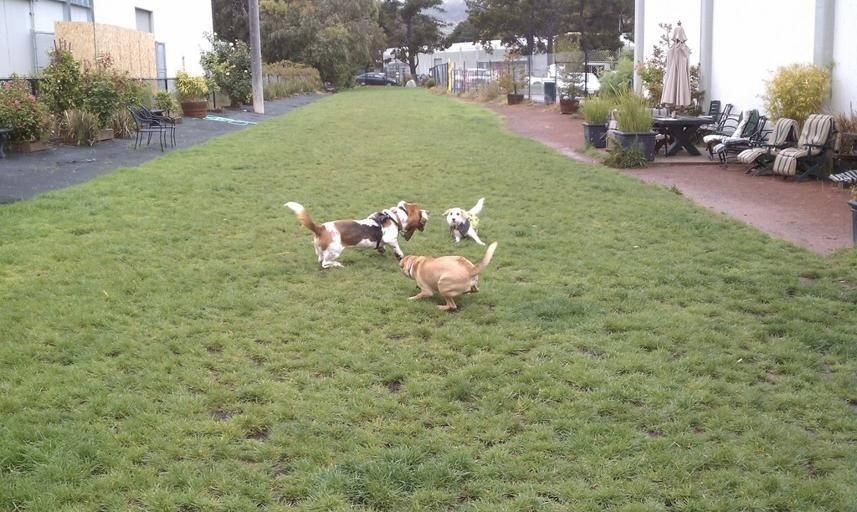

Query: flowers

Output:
[0, 72, 43, 144]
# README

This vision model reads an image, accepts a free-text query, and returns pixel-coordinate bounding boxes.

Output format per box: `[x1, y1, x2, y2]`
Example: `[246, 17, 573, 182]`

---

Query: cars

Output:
[355, 72, 399, 87]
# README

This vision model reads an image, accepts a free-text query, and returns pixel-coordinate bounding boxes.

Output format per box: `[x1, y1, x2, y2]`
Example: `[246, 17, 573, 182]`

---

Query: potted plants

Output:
[558, 84, 582, 114]
[497, 42, 530, 105]
[57, 68, 119, 145]
[579, 93, 611, 148]
[175, 70, 209, 118]
[612, 92, 655, 162]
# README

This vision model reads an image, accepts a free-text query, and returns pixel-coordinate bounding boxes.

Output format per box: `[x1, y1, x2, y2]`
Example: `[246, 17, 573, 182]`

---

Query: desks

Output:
[653, 115, 715, 156]
[0, 128, 14, 158]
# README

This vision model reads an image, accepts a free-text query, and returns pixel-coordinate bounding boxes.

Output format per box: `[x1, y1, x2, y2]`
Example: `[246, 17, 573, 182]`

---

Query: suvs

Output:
[452, 67, 490, 87]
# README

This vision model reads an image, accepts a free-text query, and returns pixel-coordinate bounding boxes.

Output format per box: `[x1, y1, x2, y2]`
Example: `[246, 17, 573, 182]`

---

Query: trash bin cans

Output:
[544, 82, 556, 106]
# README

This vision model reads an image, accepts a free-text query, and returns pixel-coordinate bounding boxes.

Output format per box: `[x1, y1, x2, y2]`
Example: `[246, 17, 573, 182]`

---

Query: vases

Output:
[10, 141, 48, 153]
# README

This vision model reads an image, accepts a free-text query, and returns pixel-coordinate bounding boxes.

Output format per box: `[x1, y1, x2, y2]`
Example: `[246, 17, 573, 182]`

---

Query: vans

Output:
[527, 62, 609, 97]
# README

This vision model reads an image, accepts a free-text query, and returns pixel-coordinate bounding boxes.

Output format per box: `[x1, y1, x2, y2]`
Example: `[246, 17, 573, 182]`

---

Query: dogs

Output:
[283, 200, 431, 269]
[441, 198, 486, 246]
[398, 241, 498, 312]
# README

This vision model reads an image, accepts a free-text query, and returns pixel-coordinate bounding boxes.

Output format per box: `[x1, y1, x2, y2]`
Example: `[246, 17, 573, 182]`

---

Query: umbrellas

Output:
[660, 21, 692, 112]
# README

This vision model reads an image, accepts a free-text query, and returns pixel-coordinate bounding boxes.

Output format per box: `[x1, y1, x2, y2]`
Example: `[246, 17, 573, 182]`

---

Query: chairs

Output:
[651, 127, 672, 158]
[126, 103, 177, 153]
[695, 100, 857, 185]
